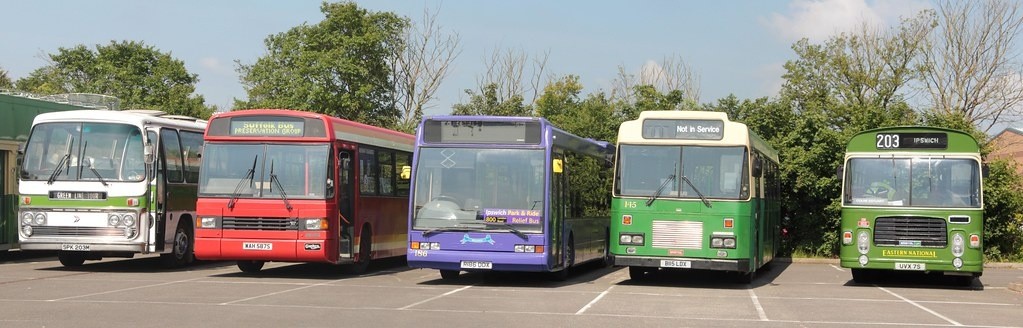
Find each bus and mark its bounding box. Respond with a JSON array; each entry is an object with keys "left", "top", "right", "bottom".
[
  {"left": 17, "top": 109, "right": 225, "bottom": 268},
  {"left": 401, "top": 116, "right": 615, "bottom": 282},
  {"left": 836, "top": 126, "right": 989, "bottom": 283},
  {"left": 194, "top": 109, "right": 416, "bottom": 275},
  {"left": 608, "top": 110, "right": 780, "bottom": 283}
]
